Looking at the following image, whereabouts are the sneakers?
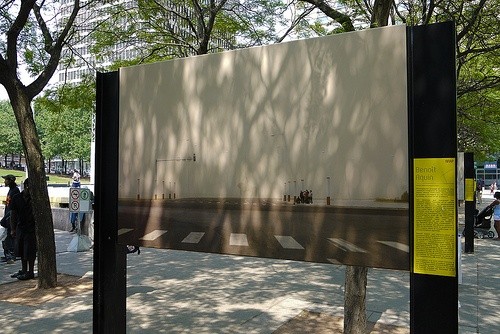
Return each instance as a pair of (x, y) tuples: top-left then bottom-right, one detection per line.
(10, 270), (34, 280)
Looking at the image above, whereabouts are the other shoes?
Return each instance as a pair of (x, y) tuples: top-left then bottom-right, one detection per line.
(7, 258), (15, 264)
(70, 227), (76, 232)
(1, 258), (8, 262)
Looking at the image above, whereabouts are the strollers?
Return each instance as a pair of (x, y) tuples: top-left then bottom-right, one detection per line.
(461, 203), (496, 239)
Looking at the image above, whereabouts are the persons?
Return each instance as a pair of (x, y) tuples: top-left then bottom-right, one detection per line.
(70, 192), (93, 233)
(476, 180), (485, 204)
(296, 189), (313, 204)
(491, 192), (500, 240)
(491, 182), (497, 193)
(0, 174), (37, 280)
(72, 169), (80, 188)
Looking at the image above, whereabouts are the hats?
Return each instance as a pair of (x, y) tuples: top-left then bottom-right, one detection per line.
(1, 174), (16, 181)
